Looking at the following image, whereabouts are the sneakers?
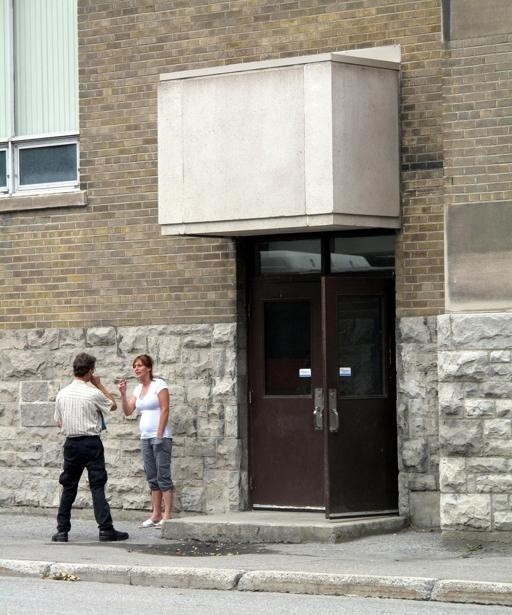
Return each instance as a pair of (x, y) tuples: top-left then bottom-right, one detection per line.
(138, 518), (166, 529)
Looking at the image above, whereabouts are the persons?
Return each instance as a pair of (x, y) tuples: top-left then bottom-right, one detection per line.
(51, 352), (130, 543)
(117, 354), (176, 530)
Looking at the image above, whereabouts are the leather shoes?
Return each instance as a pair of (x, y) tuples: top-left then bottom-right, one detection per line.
(98, 529), (131, 543)
(50, 530), (70, 542)
